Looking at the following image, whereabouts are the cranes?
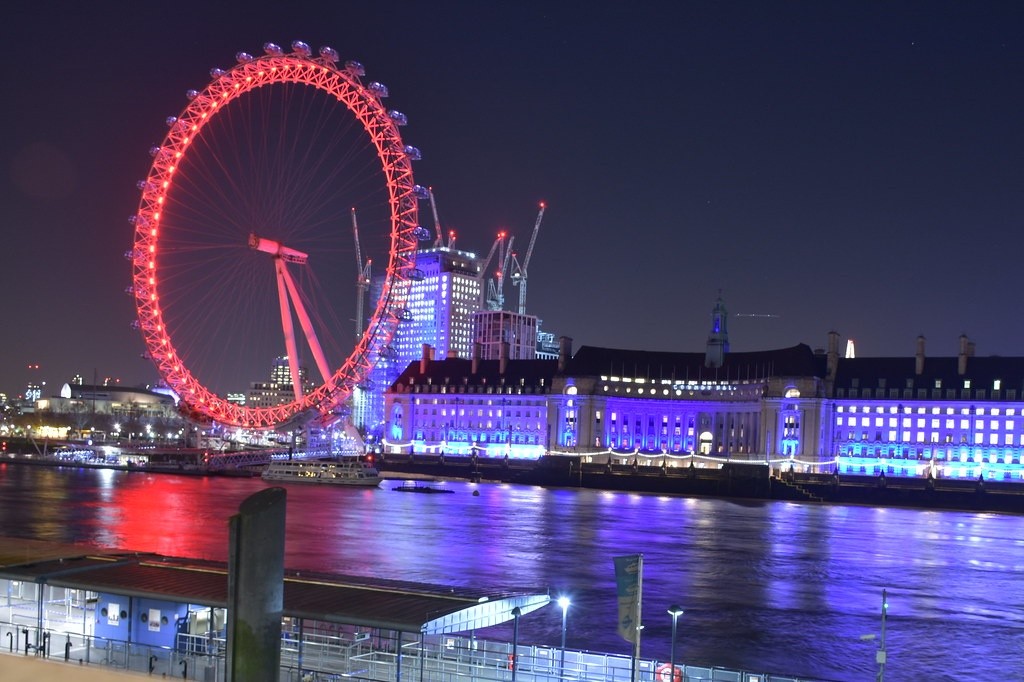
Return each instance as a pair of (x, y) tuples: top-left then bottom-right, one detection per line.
(350, 205), (372, 340)
(479, 201), (547, 314)
(429, 185), (457, 248)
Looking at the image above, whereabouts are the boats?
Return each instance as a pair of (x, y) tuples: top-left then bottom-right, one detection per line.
(260, 456), (384, 488)
(391, 480), (456, 495)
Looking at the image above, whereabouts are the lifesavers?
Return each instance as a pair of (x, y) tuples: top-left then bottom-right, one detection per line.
(656, 662), (681, 682)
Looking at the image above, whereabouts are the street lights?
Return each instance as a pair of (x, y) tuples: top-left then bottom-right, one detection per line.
(604, 445), (698, 476)
(557, 595), (573, 682)
(666, 605), (685, 682)
